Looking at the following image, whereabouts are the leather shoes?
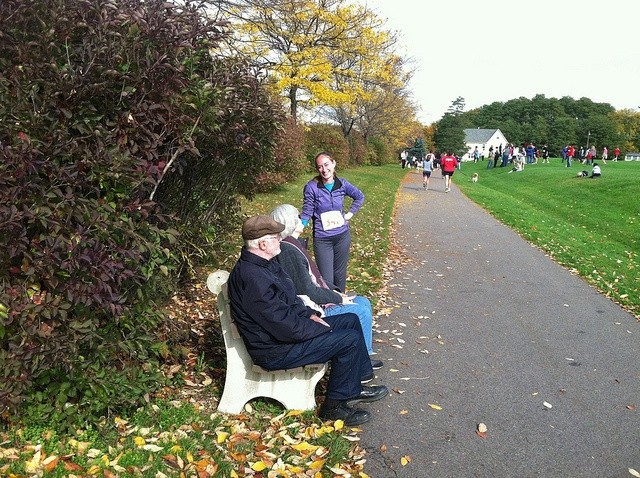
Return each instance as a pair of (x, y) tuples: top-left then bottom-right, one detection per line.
(345, 385), (388, 405)
(319, 403), (371, 426)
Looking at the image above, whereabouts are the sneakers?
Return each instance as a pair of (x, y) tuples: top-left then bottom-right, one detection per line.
(360, 373), (374, 384)
(370, 359), (383, 370)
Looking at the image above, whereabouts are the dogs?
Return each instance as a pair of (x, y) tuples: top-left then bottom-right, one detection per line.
(471, 172), (480, 183)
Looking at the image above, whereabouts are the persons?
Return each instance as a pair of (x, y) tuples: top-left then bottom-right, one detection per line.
(588, 162), (602, 178)
(487, 142), (550, 174)
(441, 151), (458, 193)
(615, 147), (620, 161)
(401, 150), (407, 169)
(297, 151), (365, 296)
(227, 216), (390, 427)
(562, 144), (596, 167)
(601, 146), (608, 164)
(270, 204), (384, 384)
(422, 154), (434, 190)
(425, 150), (436, 177)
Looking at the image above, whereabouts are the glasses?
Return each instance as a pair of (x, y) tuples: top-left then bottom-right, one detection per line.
(297, 214), (301, 218)
(272, 234), (282, 240)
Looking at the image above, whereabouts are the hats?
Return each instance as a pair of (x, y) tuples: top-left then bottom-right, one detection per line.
(242, 216), (285, 239)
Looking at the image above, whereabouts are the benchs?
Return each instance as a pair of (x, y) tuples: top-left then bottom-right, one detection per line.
(207, 268), (329, 417)
